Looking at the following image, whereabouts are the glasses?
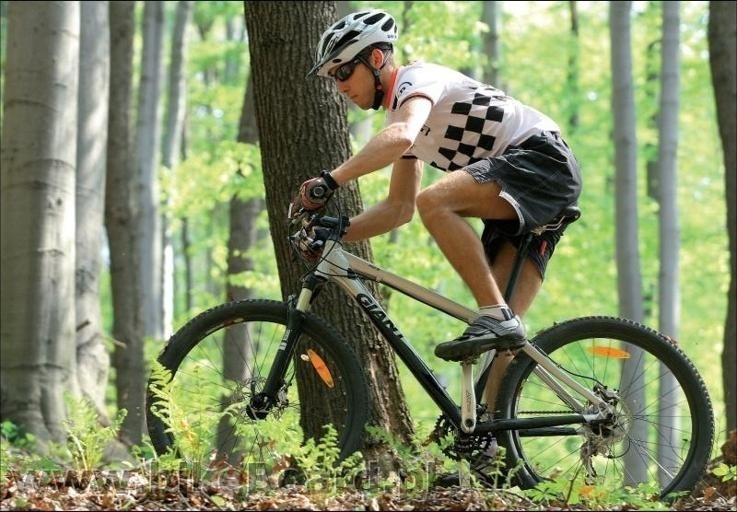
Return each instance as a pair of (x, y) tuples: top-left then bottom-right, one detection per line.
(335, 59), (360, 81)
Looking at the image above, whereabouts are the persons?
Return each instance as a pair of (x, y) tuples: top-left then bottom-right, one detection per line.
(297, 8), (585, 490)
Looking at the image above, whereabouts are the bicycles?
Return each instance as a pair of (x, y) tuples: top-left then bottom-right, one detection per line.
(145, 183), (716, 512)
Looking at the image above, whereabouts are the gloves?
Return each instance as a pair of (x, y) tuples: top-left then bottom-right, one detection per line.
(299, 239), (324, 264)
(298, 170), (340, 210)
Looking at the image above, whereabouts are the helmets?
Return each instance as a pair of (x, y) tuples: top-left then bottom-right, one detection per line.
(307, 9), (398, 77)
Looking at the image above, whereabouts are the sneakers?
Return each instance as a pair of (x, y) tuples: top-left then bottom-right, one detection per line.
(434, 314), (527, 362)
(441, 452), (517, 488)
